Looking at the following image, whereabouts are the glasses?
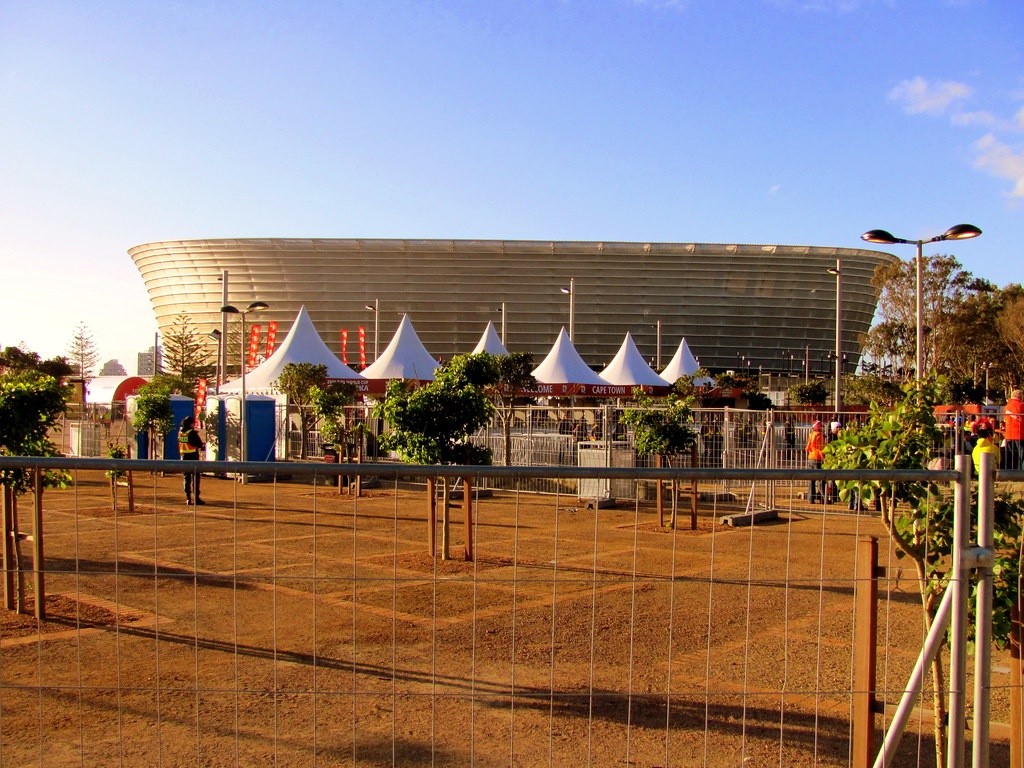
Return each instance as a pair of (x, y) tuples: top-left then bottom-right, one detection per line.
(966, 434), (971, 436)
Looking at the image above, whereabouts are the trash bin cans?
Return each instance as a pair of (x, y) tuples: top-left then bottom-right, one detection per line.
(69, 424), (101, 457)
(577, 440), (639, 502)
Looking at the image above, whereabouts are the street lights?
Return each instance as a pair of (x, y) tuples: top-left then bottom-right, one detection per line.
(208, 329), (222, 396)
(364, 304), (379, 363)
(219, 302), (269, 485)
(860, 223), (983, 408)
(560, 287), (575, 345)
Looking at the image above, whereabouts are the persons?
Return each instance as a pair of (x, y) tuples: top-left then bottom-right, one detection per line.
(176, 415), (206, 507)
(807, 389), (1024, 511)
(557, 411), (795, 465)
(101, 409), (113, 438)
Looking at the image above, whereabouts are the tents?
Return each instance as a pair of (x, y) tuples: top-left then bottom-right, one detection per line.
(598, 329), (667, 386)
(530, 325), (612, 385)
(218, 303), (368, 395)
(661, 337), (717, 388)
(471, 319), (509, 357)
(360, 312), (445, 380)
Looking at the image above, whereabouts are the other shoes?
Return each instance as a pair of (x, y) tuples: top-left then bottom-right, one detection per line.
(848, 502), (855, 510)
(820, 498), (824, 504)
(196, 500), (206, 505)
(809, 500), (816, 504)
(186, 500), (190, 505)
(856, 503), (868, 511)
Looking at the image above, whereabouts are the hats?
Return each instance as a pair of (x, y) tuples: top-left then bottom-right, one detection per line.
(831, 422), (841, 431)
(813, 420), (822, 429)
